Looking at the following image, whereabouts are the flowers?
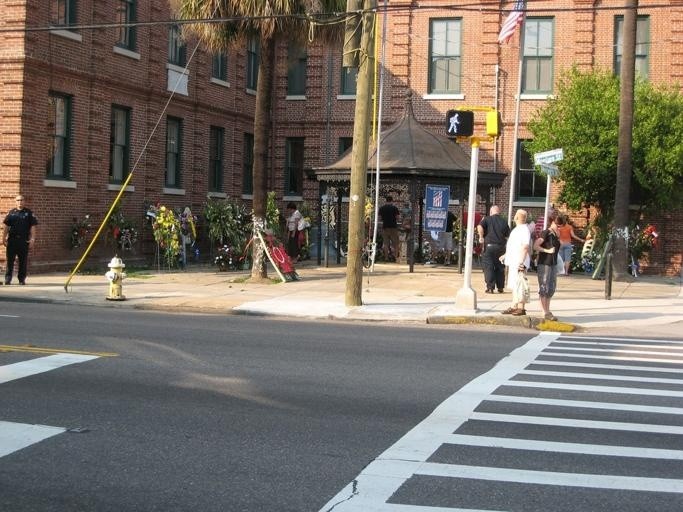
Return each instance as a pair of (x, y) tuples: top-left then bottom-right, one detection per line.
(143, 197), (201, 273)
(70, 213), (91, 249)
(201, 190), (320, 272)
(106, 203), (138, 252)
(570, 217), (661, 279)
(412, 237), (458, 266)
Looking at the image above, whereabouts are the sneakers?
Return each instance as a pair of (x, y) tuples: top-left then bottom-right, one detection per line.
(485, 288), (504, 293)
(19, 280), (25, 285)
(4, 280), (10, 285)
(545, 312), (558, 321)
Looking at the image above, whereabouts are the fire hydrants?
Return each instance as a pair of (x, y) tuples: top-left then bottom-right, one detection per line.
(105, 256), (126, 301)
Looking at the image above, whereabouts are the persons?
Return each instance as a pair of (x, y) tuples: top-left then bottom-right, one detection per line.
(476, 203), (585, 321)
(2, 195), (39, 285)
(285, 202), (305, 264)
(379, 196), (400, 262)
(425, 207), (457, 265)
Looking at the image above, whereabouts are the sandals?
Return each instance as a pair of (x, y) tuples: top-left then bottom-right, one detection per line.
(502, 307), (526, 315)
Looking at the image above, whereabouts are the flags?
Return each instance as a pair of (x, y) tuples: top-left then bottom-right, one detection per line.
(497, 0), (525, 44)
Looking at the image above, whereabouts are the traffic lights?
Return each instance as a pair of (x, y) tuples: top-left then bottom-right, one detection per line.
(445, 109), (473, 138)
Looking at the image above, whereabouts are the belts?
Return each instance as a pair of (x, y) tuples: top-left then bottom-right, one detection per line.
(487, 243), (502, 246)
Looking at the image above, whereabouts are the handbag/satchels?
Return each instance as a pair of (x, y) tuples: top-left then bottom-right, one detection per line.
(512, 271), (530, 304)
